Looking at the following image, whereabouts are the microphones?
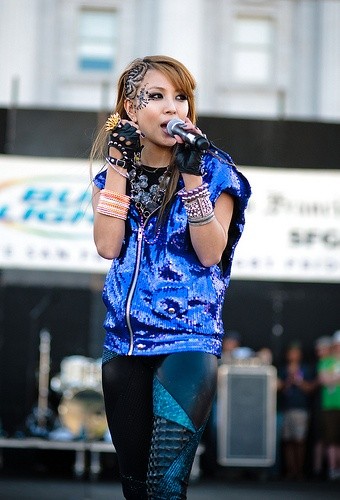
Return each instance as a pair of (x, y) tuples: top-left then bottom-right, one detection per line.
(166, 118), (209, 151)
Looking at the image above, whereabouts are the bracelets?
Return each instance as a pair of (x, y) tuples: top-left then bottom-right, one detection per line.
(176, 183), (215, 227)
(106, 158), (130, 179)
(96, 188), (130, 221)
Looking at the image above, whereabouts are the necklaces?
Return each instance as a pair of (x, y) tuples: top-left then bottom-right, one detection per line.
(130, 153), (172, 245)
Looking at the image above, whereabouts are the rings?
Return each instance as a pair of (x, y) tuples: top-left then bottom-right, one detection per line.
(105, 112), (122, 132)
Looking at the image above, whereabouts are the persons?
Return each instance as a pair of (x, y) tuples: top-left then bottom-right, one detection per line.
(45, 330), (340, 484)
(90, 56), (251, 500)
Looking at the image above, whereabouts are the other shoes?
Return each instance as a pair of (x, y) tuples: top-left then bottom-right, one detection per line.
(329, 470), (340, 480)
